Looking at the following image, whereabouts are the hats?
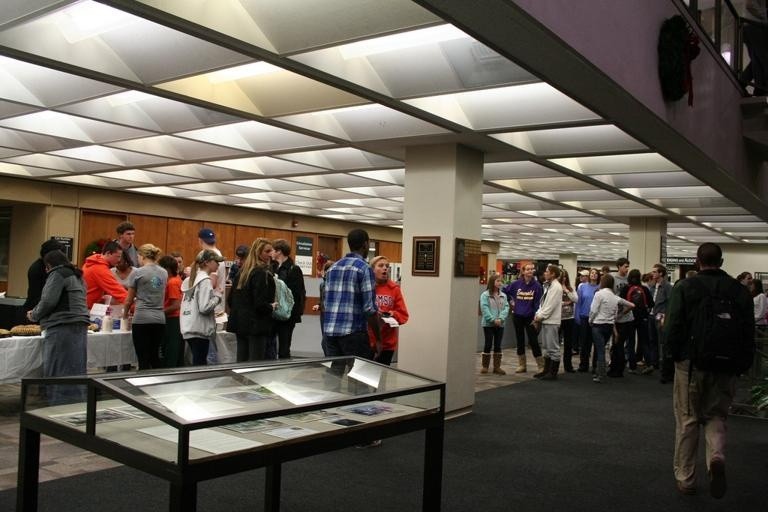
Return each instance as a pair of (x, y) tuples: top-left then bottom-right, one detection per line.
(196, 249), (226, 263)
(199, 229), (216, 243)
(579, 270), (590, 276)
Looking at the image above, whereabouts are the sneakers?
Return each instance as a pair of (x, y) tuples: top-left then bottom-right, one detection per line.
(579, 366), (588, 371)
(629, 369), (643, 375)
(565, 367), (574, 372)
(642, 366), (658, 374)
(356, 439), (380, 450)
(609, 371), (621, 378)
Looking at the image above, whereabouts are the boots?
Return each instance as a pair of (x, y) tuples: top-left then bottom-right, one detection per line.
(534, 356), (545, 373)
(540, 359), (560, 379)
(516, 354), (527, 372)
(533, 358), (553, 378)
(593, 363), (600, 379)
(593, 361), (608, 384)
(493, 354), (506, 374)
(480, 353), (490, 373)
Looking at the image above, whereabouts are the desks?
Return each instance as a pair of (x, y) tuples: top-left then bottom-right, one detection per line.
(1, 334), (236, 388)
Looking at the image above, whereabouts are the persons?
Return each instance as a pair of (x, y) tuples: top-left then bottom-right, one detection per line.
(80, 241), (136, 313)
(115, 222), (138, 270)
(481, 275), (510, 375)
(270, 240), (306, 358)
(576, 270), (590, 290)
(620, 270), (655, 374)
(642, 272), (656, 289)
(117, 243), (168, 371)
(601, 265), (610, 275)
(504, 263), (546, 373)
(159, 255), (183, 368)
(170, 252), (191, 280)
(558, 268), (578, 373)
(611, 258), (643, 375)
(575, 268), (601, 372)
(662, 243), (756, 498)
(589, 273), (635, 384)
(22, 241), (67, 309)
(184, 228), (227, 365)
(368, 256), (410, 365)
(737, 272), (768, 326)
(686, 271), (697, 279)
(535, 265), (563, 380)
(180, 249), (224, 364)
(226, 237), (279, 363)
(26, 249), (91, 404)
(109, 250), (137, 305)
(227, 244), (250, 283)
(320, 230), (382, 449)
(649, 264), (673, 330)
(311, 260), (335, 337)
(740, 0), (768, 96)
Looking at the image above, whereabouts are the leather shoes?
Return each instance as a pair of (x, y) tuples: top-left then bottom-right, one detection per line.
(677, 479), (699, 495)
(709, 458), (727, 499)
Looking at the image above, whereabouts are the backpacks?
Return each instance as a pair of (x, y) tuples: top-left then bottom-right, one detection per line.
(272, 272), (294, 321)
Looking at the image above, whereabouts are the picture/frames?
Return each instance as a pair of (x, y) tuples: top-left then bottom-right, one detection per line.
(412, 236), (441, 277)
